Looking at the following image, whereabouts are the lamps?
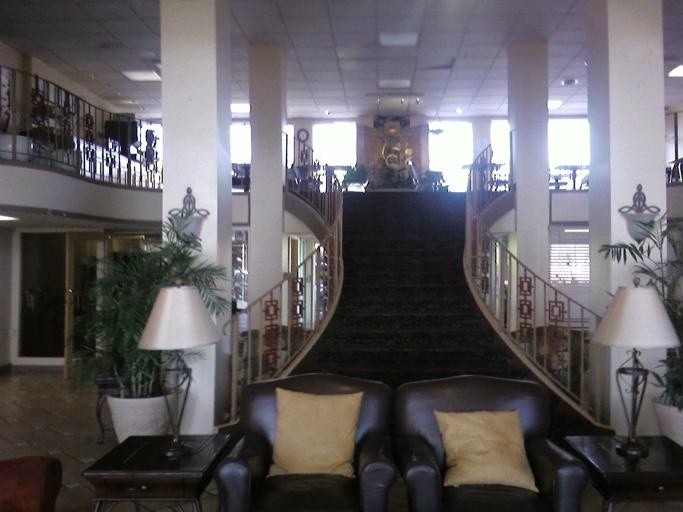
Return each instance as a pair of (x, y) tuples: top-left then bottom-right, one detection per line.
(592, 278), (680, 460)
(617, 181), (662, 246)
(137, 274), (221, 461)
(165, 184), (213, 247)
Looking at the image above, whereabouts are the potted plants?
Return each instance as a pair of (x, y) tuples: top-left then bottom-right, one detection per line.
(59, 216), (231, 447)
(598, 206), (682, 447)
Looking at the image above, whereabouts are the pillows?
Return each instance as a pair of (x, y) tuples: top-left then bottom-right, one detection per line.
(268, 388), (364, 480)
(434, 409), (540, 494)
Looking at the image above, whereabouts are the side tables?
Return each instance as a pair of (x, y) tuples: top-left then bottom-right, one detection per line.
(563, 430), (681, 512)
(81, 432), (235, 510)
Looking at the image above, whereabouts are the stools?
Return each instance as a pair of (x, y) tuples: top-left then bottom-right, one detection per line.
(0, 452), (64, 510)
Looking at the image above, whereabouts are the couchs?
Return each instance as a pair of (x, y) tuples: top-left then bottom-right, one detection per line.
(395, 375), (590, 511)
(216, 374), (394, 510)
